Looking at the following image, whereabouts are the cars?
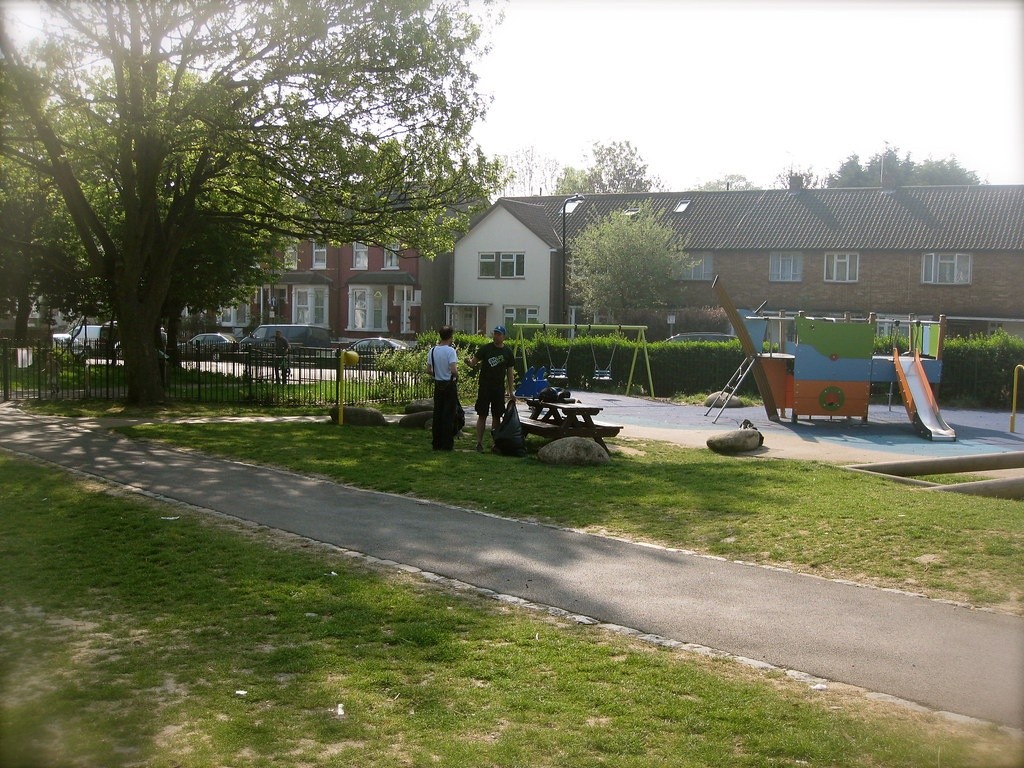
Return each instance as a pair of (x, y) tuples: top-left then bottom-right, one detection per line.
(113, 331), (167, 360)
(53, 333), (86, 360)
(176, 333), (238, 362)
(337, 337), (416, 372)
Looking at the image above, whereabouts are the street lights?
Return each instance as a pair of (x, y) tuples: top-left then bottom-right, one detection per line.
(563, 195), (586, 340)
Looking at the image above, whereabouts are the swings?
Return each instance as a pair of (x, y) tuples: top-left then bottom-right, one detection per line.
(590, 330), (620, 380)
(543, 331), (575, 380)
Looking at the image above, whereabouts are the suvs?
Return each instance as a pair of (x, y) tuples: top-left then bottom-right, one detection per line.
(660, 332), (738, 345)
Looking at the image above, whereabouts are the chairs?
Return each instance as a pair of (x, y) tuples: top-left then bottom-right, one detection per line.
(548, 368), (567, 378)
(593, 370), (613, 380)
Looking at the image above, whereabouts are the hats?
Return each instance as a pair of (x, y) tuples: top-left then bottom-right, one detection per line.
(493, 325), (506, 336)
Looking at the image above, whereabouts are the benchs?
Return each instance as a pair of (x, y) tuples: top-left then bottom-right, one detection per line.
(519, 417), (561, 441)
(562, 415), (624, 438)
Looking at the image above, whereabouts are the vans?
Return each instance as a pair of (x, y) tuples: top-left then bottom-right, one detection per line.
(238, 325), (338, 358)
(65, 324), (118, 360)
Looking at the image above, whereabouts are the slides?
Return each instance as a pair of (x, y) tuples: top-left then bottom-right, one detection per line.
(892, 348), (956, 441)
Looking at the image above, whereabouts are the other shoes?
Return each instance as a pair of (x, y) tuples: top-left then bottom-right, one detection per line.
(478, 444), (485, 454)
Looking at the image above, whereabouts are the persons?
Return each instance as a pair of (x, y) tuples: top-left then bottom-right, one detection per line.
(274, 330), (290, 386)
(161, 327), (167, 351)
(464, 326), (516, 454)
(425, 325), (460, 452)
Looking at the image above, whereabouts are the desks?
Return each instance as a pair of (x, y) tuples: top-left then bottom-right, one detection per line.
(526, 398), (610, 455)
